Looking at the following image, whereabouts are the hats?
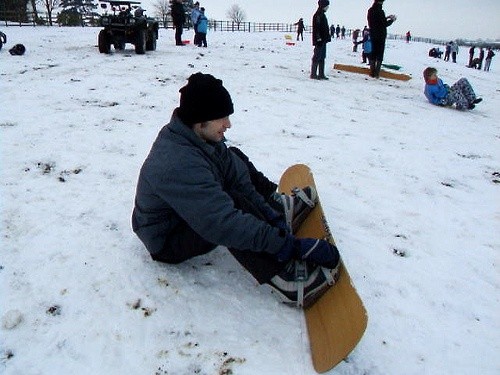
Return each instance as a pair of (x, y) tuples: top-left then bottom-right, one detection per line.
(179, 73), (234, 123)
(319, 0), (329, 8)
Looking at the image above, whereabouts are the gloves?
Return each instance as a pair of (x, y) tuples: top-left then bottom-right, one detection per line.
(265, 207), (290, 233)
(280, 235), (340, 268)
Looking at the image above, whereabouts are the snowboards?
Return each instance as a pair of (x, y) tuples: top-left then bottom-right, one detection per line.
(279, 163), (368, 372)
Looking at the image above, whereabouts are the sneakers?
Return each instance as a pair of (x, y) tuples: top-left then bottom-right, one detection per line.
(270, 185), (316, 234)
(264, 260), (340, 308)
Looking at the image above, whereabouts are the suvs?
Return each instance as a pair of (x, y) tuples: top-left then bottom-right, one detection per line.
(98, 0), (158, 55)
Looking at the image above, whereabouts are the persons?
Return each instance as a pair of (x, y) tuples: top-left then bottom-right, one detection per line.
(406, 30), (411, 43)
(353, 29), (373, 65)
(423, 67), (482, 110)
(477, 47), (484, 69)
(362, 26), (368, 49)
(190, 2), (200, 44)
(469, 46), (476, 66)
(429, 48), (437, 58)
(352, 29), (360, 52)
(132, 72), (341, 310)
(484, 46), (495, 71)
(341, 26), (345, 39)
(294, 18), (305, 41)
(194, 7), (208, 47)
(444, 42), (451, 62)
(434, 48), (441, 58)
(310, 0), (331, 80)
(449, 41), (459, 63)
(336, 24), (340, 37)
(468, 58), (480, 69)
(330, 25), (335, 38)
(171, 0), (186, 45)
